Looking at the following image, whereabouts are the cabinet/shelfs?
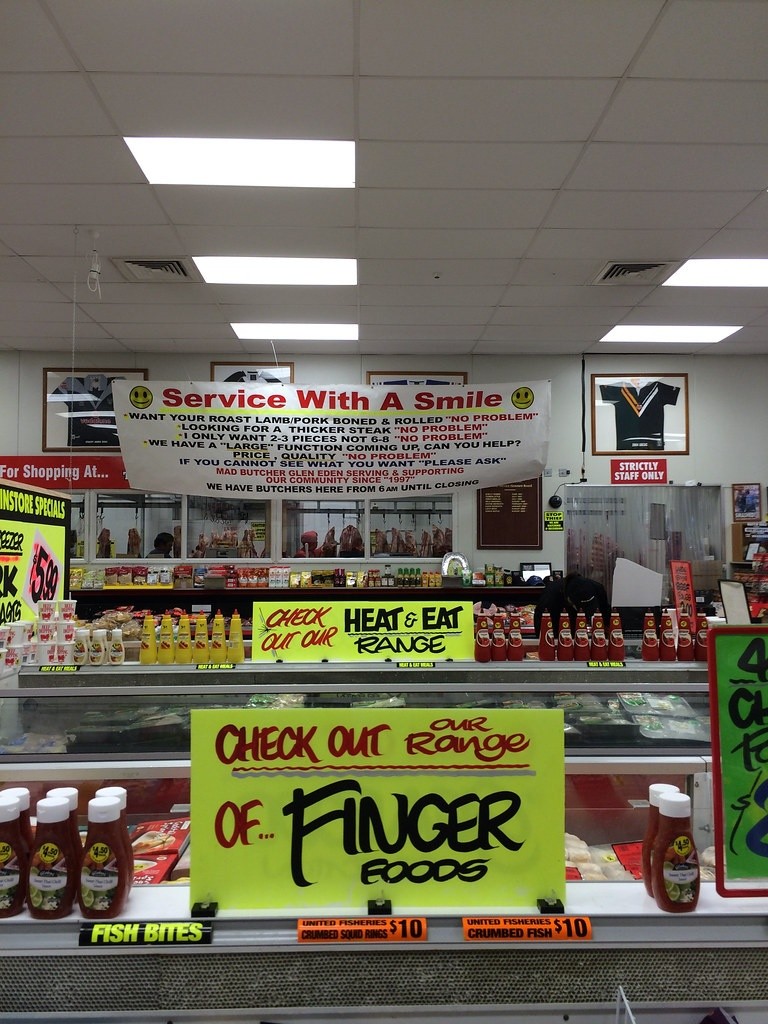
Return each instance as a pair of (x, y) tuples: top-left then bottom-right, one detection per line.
(731, 522), (768, 562)
(0, 662), (768, 1024)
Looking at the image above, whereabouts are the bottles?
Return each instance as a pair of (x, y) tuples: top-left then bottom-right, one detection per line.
(227, 609), (245, 663)
(268, 565), (290, 587)
(539, 607), (625, 662)
(138, 609), (157, 664)
(640, 607), (723, 662)
(76, 630), (125, 665)
(157, 609), (175, 664)
(641, 781), (700, 913)
(193, 610), (209, 664)
(210, 609), (226, 663)
(333, 564), (442, 587)
(175, 609), (192, 663)
(474, 608), (524, 663)
(0, 785), (135, 920)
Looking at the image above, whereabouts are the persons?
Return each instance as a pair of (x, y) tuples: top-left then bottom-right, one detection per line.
(295, 530), (323, 557)
(735, 487), (757, 513)
(145, 532), (174, 558)
(534, 575), (610, 638)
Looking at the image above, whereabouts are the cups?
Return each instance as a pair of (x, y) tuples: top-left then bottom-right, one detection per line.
(1, 600), (77, 674)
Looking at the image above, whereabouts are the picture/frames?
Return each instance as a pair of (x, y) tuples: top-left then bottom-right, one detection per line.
(732, 483), (761, 522)
(520, 562), (552, 583)
(717, 578), (752, 624)
(41, 368), (149, 451)
(590, 373), (690, 455)
(209, 361), (294, 383)
(366, 371), (468, 385)
(744, 541), (761, 562)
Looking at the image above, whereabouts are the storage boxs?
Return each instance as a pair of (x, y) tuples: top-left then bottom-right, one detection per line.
(512, 570), (523, 585)
(462, 692), (712, 749)
(203, 577), (226, 589)
(693, 571), (726, 591)
(553, 570), (563, 582)
(472, 580), (486, 587)
(651, 539), (687, 574)
(173, 577), (193, 588)
(692, 560), (722, 576)
(205, 546), (240, 558)
(65, 708), (190, 754)
(442, 576), (463, 587)
